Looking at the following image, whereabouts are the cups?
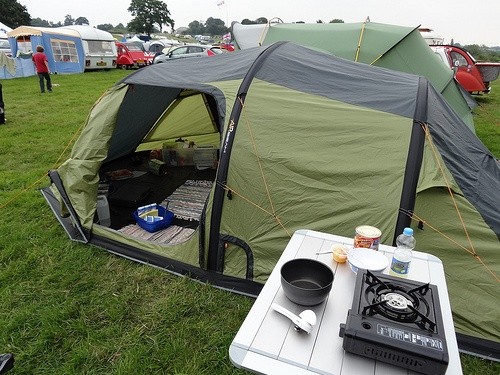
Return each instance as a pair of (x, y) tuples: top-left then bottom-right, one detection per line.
(333, 244), (348, 263)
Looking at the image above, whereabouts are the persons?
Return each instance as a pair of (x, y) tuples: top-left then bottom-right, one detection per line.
(32, 45), (53, 92)
(0, 84), (7, 124)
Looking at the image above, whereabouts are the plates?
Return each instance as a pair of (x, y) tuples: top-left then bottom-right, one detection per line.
(108, 175), (132, 180)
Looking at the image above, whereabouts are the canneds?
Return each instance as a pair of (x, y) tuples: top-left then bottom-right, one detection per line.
(352, 225), (382, 251)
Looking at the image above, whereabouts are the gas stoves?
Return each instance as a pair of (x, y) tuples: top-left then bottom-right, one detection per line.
(339, 269), (449, 375)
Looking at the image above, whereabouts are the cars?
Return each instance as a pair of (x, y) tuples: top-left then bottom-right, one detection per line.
(153, 44), (223, 64)
(0, 21), (211, 72)
(428, 44), (500, 96)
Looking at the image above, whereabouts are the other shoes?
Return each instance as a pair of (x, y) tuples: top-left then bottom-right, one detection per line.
(47, 88), (52, 91)
(0, 119), (7, 124)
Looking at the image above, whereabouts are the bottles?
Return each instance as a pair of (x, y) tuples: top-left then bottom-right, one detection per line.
(162, 142), (178, 167)
(147, 158), (168, 176)
(389, 227), (417, 279)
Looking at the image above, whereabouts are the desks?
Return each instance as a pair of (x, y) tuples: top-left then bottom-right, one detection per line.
(228, 228), (462, 375)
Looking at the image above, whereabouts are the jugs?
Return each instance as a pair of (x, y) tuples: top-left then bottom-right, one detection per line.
(96, 195), (111, 227)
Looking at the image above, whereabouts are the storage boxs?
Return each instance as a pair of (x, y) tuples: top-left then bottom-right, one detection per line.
(133, 204), (174, 232)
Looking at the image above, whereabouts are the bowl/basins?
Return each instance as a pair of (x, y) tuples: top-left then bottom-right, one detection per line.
(132, 205), (174, 233)
(280, 258), (335, 305)
(346, 248), (389, 274)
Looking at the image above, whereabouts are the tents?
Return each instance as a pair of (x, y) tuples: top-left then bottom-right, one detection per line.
(0, 22), (12, 56)
(40, 40), (500, 362)
(458, 83), (482, 107)
(126, 35), (145, 43)
(7, 26), (85, 75)
(231, 18), (478, 134)
